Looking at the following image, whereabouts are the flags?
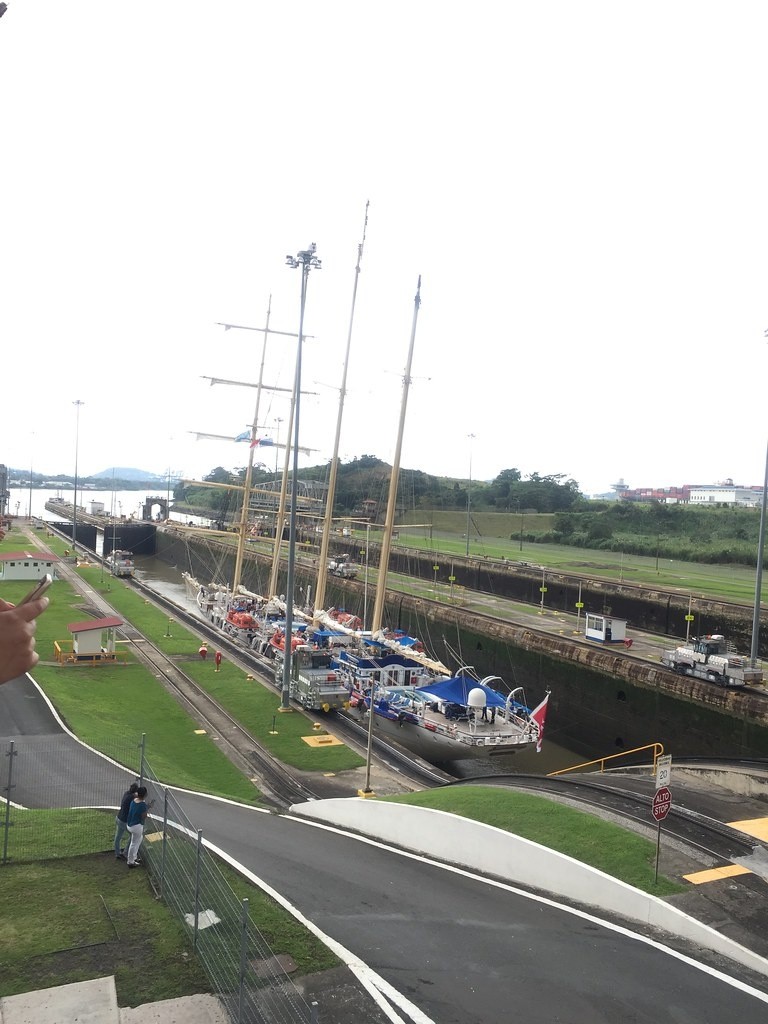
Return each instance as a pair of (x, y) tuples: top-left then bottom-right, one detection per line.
(232, 430), (274, 449)
(529, 694), (550, 753)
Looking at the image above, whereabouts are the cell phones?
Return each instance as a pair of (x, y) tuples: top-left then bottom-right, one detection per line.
(17, 574), (53, 607)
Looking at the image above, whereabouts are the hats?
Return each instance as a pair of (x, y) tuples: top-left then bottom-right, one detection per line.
(469, 707), (473, 710)
(138, 787), (148, 799)
(129, 783), (139, 793)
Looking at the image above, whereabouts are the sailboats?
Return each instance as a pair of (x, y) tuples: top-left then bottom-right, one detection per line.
(180, 196), (551, 761)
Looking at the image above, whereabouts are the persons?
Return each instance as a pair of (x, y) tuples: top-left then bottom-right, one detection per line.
(481, 705), (496, 725)
(115, 783), (154, 868)
(0, 597), (49, 685)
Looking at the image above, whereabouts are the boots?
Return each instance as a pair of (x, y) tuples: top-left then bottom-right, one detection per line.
(489, 719), (495, 724)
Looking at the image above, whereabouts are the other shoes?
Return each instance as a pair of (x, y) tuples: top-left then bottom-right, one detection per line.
(401, 725), (404, 728)
(116, 853), (128, 862)
(466, 718), (475, 723)
(481, 716), (489, 722)
(128, 859), (141, 867)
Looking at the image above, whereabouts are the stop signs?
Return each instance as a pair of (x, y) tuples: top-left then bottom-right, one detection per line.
(652, 789), (672, 820)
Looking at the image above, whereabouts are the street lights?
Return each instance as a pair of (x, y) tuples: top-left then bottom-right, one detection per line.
(465, 434), (478, 557)
(72, 399), (85, 549)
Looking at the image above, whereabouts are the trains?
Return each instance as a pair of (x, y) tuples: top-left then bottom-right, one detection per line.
(327, 553), (358, 579)
(661, 635), (764, 688)
(272, 644), (352, 713)
(110, 550), (136, 577)
